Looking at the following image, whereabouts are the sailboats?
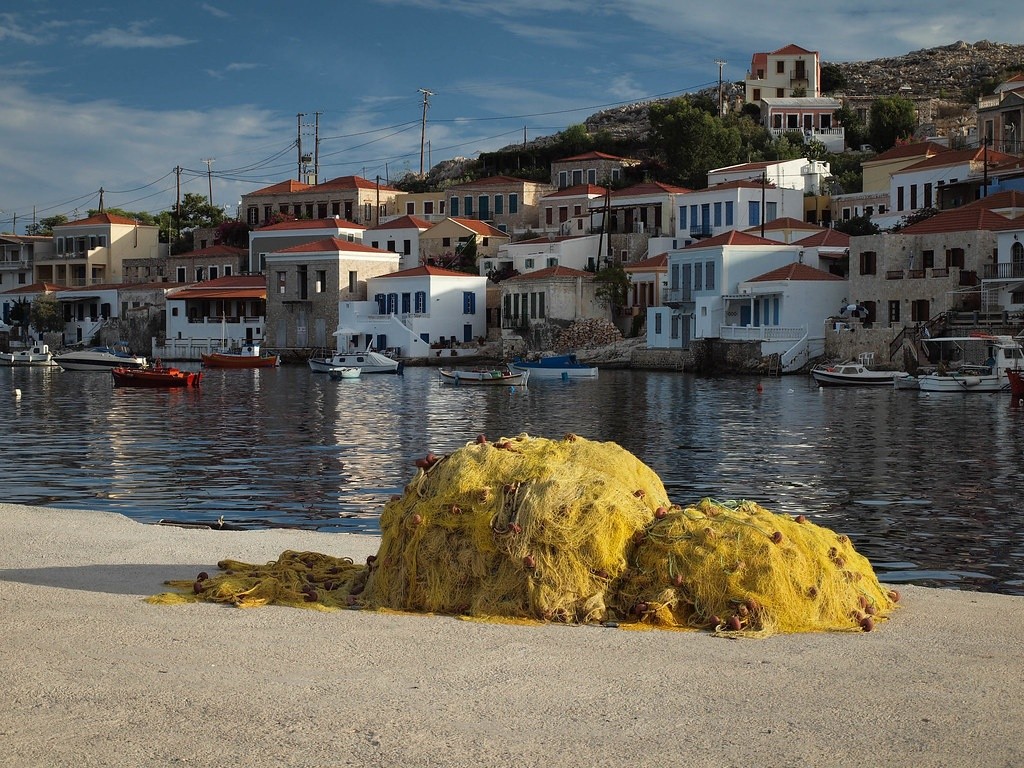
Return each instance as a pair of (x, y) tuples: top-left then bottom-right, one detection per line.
(201, 311), (277, 368)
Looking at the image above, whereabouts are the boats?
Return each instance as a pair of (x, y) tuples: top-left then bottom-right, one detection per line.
(439, 368), (526, 386)
(327, 367), (361, 378)
(111, 358), (203, 388)
(506, 354), (598, 380)
(917, 344), (1024, 392)
(1004, 368), (1024, 397)
(308, 351), (399, 373)
(810, 365), (909, 387)
(51, 347), (147, 371)
(0, 341), (58, 366)
